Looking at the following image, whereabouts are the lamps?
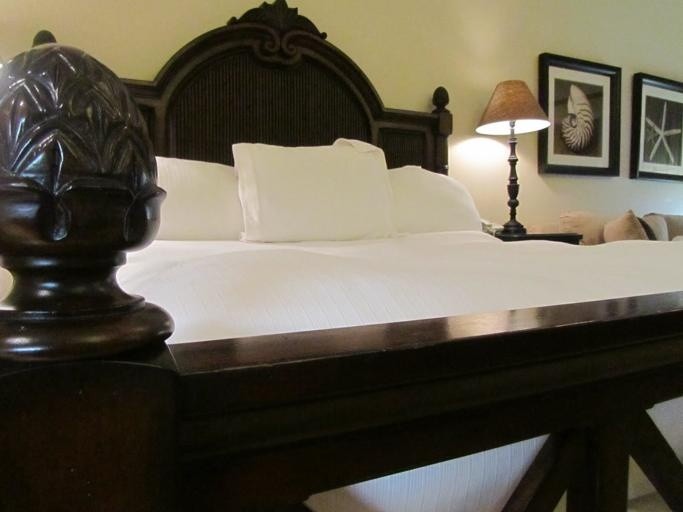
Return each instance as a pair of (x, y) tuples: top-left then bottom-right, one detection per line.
(475, 80), (551, 234)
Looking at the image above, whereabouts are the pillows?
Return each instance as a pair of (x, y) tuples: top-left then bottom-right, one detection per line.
(154, 156), (244, 241)
(231, 139), (410, 243)
(387, 165), (481, 235)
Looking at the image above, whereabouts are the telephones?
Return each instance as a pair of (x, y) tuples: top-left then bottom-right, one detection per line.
(481, 219), (504, 235)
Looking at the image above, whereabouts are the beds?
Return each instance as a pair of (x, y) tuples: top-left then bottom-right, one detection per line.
(0, 0), (683, 511)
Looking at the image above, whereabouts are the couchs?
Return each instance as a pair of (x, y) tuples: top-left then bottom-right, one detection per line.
(558, 211), (683, 246)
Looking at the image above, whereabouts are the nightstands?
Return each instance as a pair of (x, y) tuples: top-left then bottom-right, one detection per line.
(493, 232), (582, 246)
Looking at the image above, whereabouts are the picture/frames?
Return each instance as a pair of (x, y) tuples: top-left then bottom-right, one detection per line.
(538, 53), (683, 181)
